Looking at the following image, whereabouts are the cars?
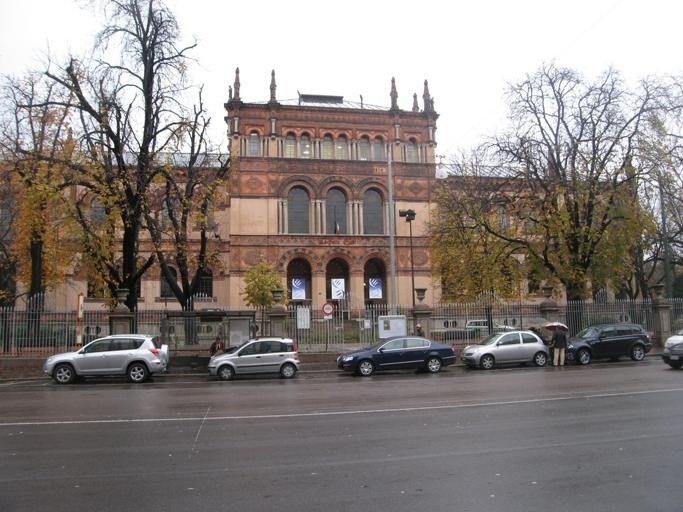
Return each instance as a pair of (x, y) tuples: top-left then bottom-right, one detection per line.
(465, 320), (514, 336)
(207, 337), (300, 380)
(336, 336), (457, 376)
(549, 323), (652, 365)
(460, 330), (550, 370)
(662, 329), (683, 368)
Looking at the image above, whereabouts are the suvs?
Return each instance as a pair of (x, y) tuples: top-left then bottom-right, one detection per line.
(42, 334), (168, 384)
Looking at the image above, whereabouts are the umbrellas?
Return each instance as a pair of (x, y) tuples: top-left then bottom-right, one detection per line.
(542, 319), (568, 331)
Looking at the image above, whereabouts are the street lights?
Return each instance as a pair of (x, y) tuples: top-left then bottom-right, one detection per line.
(399, 209), (416, 305)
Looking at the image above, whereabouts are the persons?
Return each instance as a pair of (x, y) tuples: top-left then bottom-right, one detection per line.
(413, 323), (425, 337)
(550, 325), (567, 367)
(209, 336), (224, 356)
